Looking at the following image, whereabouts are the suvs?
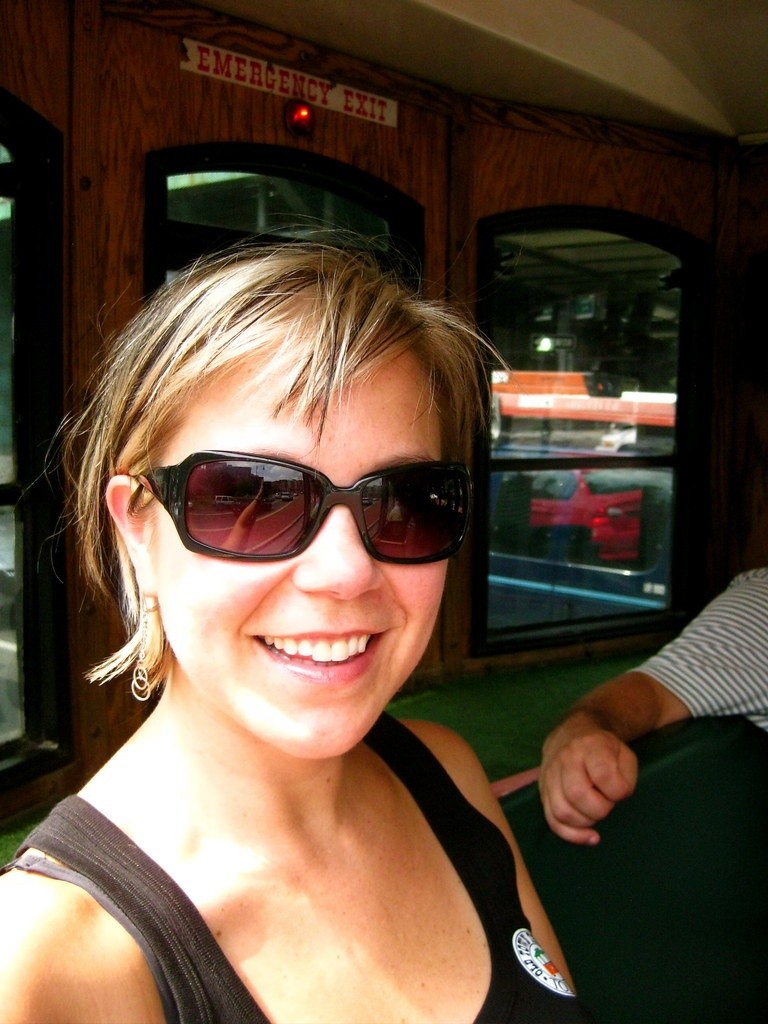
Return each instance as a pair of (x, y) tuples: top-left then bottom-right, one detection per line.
(214, 496), (242, 511)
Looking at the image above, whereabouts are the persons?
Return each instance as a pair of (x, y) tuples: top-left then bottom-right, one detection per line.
(0, 242), (588, 1024)
(222, 478), (266, 553)
(534, 568), (768, 845)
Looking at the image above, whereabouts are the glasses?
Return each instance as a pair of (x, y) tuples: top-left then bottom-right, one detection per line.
(129, 448), (476, 564)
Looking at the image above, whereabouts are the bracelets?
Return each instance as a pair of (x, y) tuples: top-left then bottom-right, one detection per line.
(254, 495), (262, 502)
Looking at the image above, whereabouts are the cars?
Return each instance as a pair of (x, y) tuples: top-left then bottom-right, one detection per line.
(492, 447), (645, 565)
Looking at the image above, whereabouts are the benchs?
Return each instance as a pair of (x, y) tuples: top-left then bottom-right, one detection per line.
(387, 644), (768, 1024)
